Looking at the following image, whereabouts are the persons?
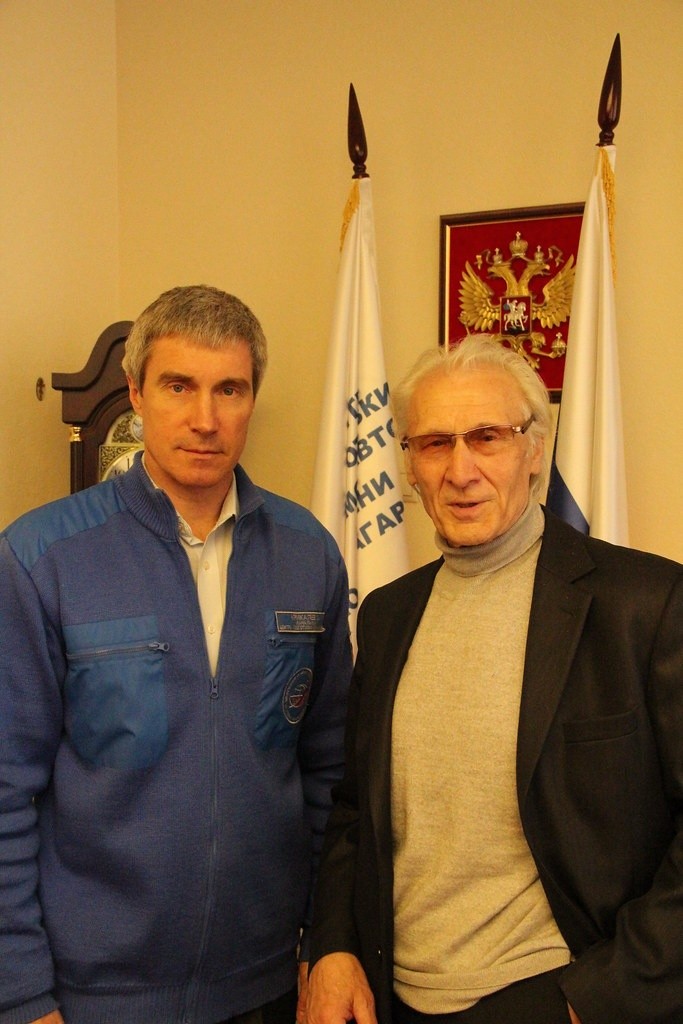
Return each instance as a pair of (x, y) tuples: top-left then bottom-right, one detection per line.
(0, 284), (358, 1024)
(301, 326), (682, 1024)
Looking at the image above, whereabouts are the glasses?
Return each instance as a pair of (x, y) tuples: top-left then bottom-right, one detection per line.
(401, 414), (536, 455)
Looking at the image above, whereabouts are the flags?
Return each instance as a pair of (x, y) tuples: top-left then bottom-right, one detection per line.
(541, 152), (643, 553)
(310, 176), (410, 670)
